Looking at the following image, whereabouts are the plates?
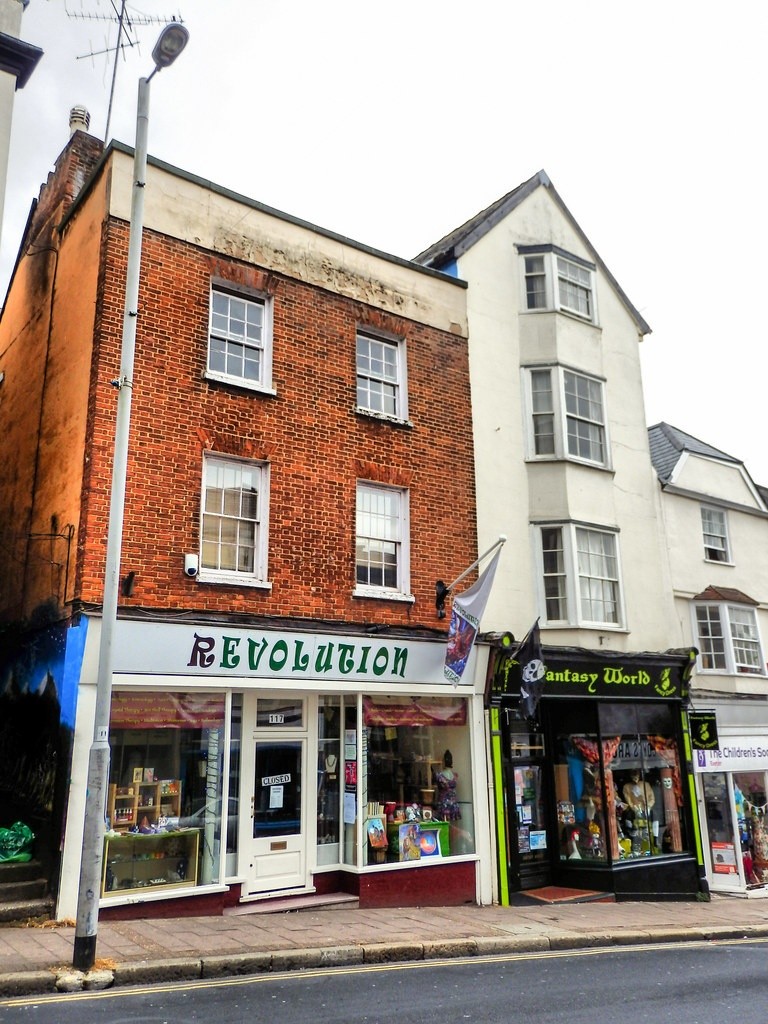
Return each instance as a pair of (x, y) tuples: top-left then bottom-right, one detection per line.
(149, 878), (166, 884)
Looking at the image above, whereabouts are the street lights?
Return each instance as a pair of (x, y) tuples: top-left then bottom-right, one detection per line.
(69, 21), (193, 971)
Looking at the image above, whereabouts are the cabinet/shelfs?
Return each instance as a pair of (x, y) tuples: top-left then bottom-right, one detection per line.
(368, 721), (444, 809)
(99, 825), (202, 899)
(106, 778), (184, 835)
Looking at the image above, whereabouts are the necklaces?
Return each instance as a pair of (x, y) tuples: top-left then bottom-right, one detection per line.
(326, 758), (335, 767)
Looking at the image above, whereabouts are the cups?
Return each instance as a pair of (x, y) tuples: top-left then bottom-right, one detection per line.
(138, 795), (144, 806)
(147, 798), (153, 806)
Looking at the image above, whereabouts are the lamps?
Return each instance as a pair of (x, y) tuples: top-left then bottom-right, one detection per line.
(476, 630), (516, 649)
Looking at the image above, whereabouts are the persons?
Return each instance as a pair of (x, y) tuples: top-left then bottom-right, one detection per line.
(623, 770), (655, 839)
(436, 766), (462, 822)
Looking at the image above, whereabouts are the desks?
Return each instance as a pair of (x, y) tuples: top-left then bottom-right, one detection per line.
(384, 820), (450, 862)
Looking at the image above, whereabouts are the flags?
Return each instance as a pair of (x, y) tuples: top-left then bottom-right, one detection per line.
(444, 544), (503, 688)
(511, 621), (548, 721)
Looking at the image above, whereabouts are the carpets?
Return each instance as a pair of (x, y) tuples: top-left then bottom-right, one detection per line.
(518, 885), (606, 904)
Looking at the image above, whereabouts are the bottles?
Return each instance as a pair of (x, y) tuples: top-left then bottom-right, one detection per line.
(115, 808), (133, 821)
